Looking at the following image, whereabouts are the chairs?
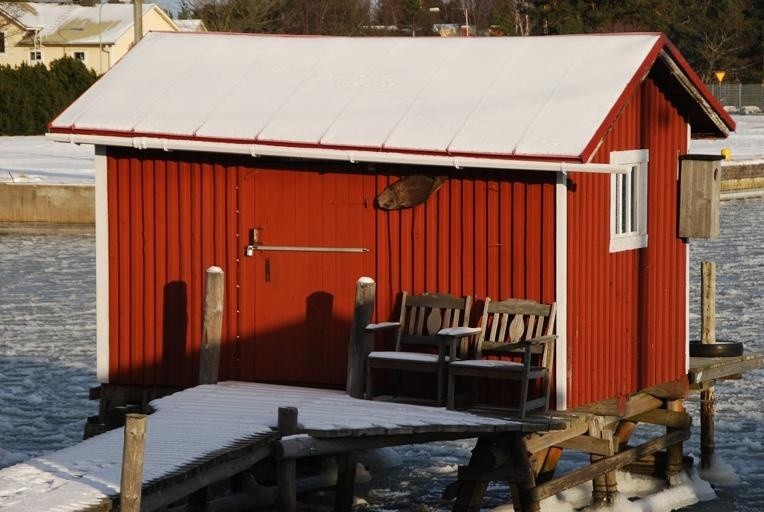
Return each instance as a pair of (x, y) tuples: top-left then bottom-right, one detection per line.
(364, 289), (557, 418)
